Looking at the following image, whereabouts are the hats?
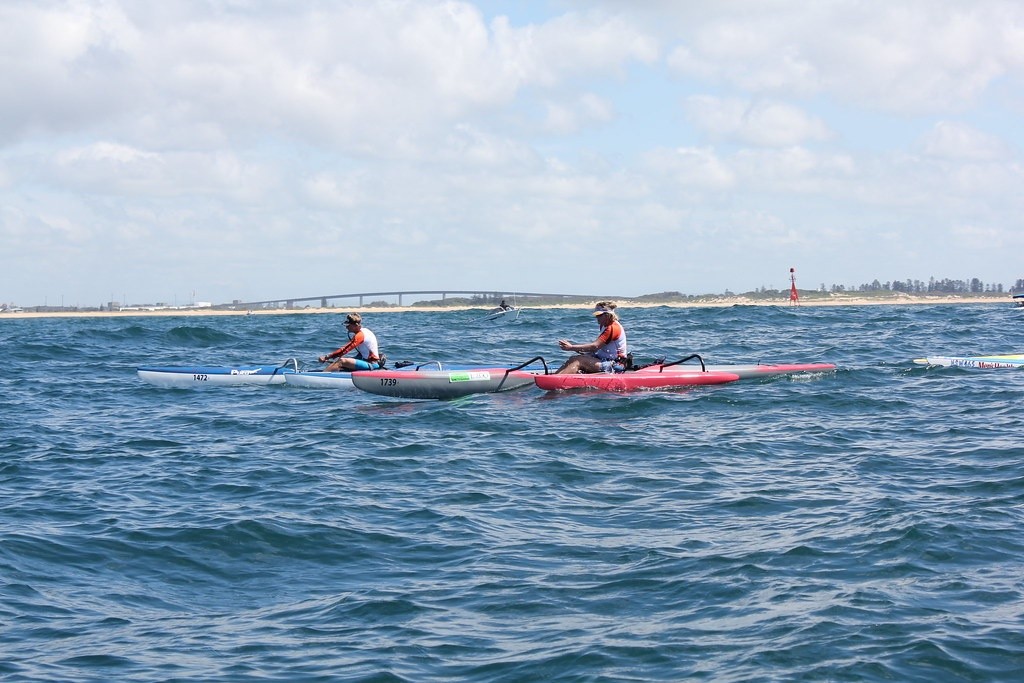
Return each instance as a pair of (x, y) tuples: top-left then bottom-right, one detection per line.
(592, 304), (614, 316)
(343, 318), (360, 324)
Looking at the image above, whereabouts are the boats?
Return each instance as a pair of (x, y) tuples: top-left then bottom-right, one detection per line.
(351, 360), (539, 398)
(535, 354), (739, 391)
(135, 357), (301, 386)
(914, 353), (1024, 369)
(1008, 294), (1024, 312)
(283, 371), (357, 390)
(471, 305), (523, 323)
(641, 361), (836, 380)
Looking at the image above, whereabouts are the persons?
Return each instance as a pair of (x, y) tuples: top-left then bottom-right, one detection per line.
(500, 300), (511, 311)
(319, 313), (380, 372)
(553, 302), (628, 374)
